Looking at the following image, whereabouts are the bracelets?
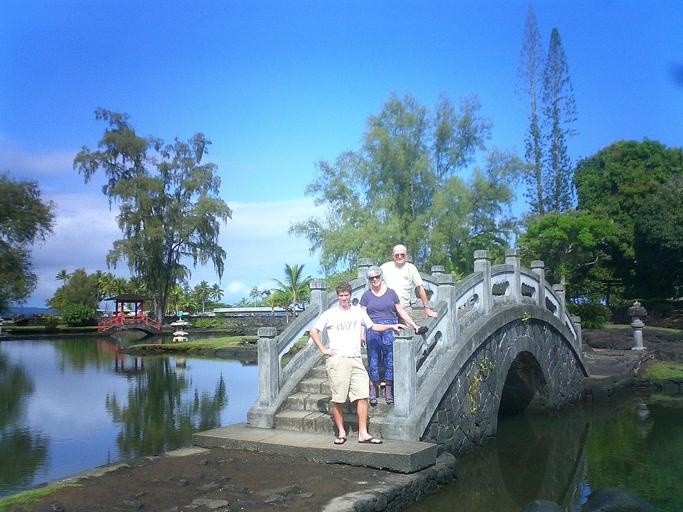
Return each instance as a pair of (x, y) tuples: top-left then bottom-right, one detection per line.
(424, 305), (430, 308)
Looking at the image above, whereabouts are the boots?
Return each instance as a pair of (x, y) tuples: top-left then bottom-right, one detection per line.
(368, 381), (379, 406)
(385, 380), (394, 403)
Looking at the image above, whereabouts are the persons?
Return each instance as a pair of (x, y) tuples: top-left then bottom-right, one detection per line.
(357, 265), (424, 406)
(307, 280), (408, 445)
(377, 244), (439, 329)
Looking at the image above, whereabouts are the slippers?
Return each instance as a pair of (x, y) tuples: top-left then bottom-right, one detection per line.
(333, 436), (347, 444)
(357, 437), (382, 445)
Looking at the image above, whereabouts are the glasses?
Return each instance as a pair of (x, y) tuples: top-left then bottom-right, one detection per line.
(394, 253), (406, 259)
(368, 274), (379, 281)
